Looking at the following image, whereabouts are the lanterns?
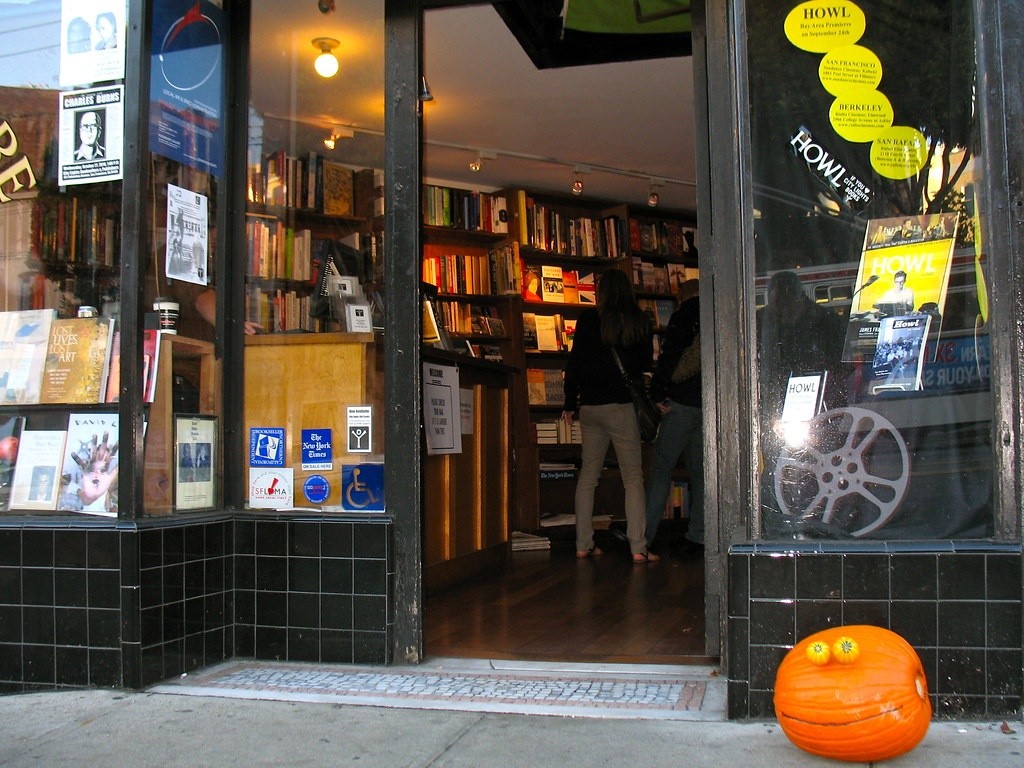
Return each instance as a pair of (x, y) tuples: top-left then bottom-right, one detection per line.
(773, 623), (932, 762)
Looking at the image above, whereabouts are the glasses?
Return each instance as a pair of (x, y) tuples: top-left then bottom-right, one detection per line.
(79, 123), (101, 130)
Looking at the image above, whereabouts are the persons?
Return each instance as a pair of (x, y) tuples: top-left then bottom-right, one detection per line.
(758, 271), (847, 526)
(180, 443), (210, 482)
(864, 211), (951, 379)
(29, 465), (54, 500)
(560, 268), (663, 563)
(609, 279), (705, 546)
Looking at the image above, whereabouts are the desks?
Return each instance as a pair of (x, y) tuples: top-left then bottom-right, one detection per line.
(838, 393), (991, 527)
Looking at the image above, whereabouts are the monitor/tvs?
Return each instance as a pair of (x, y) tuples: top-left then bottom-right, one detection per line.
(308, 239), (372, 319)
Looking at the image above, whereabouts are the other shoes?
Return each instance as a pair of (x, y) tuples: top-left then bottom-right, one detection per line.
(607, 523), (630, 542)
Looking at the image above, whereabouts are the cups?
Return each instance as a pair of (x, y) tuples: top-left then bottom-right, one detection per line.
(77, 306), (99, 319)
(151, 294), (180, 336)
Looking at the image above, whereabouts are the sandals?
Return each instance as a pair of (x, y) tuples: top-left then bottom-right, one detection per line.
(633, 550), (662, 562)
(577, 544), (606, 556)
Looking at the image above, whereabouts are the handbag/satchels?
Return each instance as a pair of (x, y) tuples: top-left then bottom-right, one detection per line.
(670, 334), (700, 383)
(627, 376), (663, 444)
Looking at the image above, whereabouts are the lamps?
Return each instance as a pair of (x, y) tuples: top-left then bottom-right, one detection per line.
(310, 38), (340, 78)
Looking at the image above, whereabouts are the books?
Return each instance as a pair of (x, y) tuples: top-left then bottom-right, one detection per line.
(781, 370), (828, 436)
(8, 89), (777, 459)
(512, 464), (692, 552)
(868, 314), (933, 395)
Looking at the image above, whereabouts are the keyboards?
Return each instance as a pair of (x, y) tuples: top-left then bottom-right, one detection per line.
(283, 329), (317, 333)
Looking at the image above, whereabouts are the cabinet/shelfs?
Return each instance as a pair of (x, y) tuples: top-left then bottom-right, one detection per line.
(238, 335), (522, 592)
(0, 113), (701, 516)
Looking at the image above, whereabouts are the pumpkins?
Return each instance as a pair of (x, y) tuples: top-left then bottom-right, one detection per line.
(774, 625), (931, 761)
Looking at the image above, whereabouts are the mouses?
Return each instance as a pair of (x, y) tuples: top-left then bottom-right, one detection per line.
(255, 328), (268, 334)
